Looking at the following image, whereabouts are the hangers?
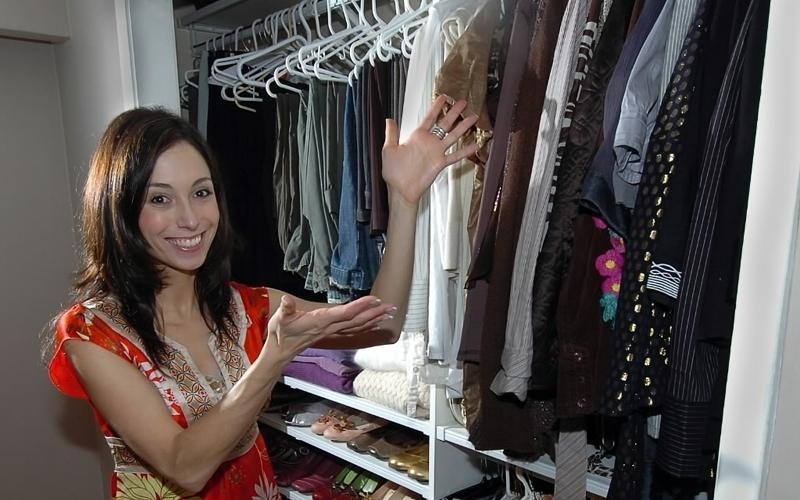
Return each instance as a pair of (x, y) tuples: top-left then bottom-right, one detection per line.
(181, 0), (465, 114)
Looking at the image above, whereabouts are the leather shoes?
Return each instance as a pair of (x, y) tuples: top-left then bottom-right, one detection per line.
(283, 400), (337, 427)
(387, 441), (430, 473)
(310, 408), (347, 437)
(274, 450), (323, 488)
(366, 428), (425, 464)
(346, 424), (404, 452)
(322, 417), (387, 443)
(408, 460), (429, 483)
(282, 395), (320, 420)
(290, 461), (340, 493)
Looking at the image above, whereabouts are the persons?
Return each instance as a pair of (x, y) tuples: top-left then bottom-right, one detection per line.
(47, 93), (481, 499)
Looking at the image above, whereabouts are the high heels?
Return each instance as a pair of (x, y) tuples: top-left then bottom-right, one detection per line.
(388, 487), (415, 500)
(368, 479), (395, 500)
(332, 471), (385, 499)
(312, 463), (361, 500)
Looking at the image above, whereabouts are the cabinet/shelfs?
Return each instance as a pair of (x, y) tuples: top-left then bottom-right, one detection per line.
(171, 0), (799, 499)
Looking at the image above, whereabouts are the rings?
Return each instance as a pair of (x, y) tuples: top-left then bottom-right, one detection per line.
(432, 127), (446, 140)
